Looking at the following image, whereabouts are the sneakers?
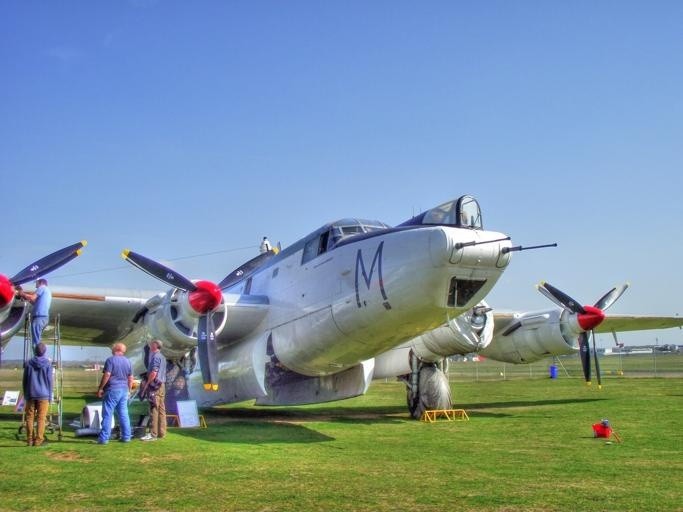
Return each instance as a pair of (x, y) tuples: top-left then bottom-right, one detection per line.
(140, 434), (157, 442)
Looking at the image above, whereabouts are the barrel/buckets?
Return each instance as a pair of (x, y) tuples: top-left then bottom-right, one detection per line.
(593, 423), (613, 437)
(551, 366), (558, 378)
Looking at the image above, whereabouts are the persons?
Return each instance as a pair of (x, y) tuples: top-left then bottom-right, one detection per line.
(138, 339), (167, 441)
(18, 278), (51, 355)
(95, 343), (133, 445)
(259, 236), (271, 253)
(167, 375), (188, 414)
(21, 343), (53, 446)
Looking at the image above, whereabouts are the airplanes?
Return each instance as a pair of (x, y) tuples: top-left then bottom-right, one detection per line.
(0, 196), (681, 419)
